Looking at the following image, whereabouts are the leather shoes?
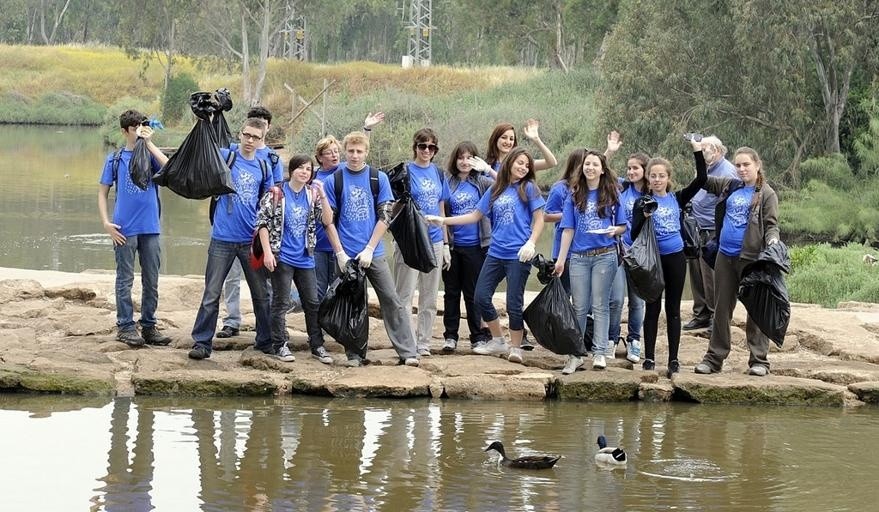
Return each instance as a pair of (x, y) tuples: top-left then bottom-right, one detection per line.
(683, 317), (711, 331)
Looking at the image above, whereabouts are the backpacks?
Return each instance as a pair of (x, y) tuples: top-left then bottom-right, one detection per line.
(209, 149), (267, 226)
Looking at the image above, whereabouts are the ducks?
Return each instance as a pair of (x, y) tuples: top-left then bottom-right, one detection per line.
(484, 441), (561, 469)
(594, 436), (627, 465)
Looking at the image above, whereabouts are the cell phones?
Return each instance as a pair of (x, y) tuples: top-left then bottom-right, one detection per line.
(685, 133), (703, 142)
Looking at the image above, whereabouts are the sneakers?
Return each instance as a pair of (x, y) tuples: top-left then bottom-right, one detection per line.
(404, 345), (431, 367)
(442, 333), (535, 365)
(560, 350), (608, 374)
(188, 343), (212, 359)
(310, 346), (334, 365)
(626, 339), (658, 371)
(694, 362), (712, 375)
(748, 363), (768, 376)
(667, 360), (679, 379)
(257, 344), (296, 362)
(216, 325), (240, 338)
(142, 326), (173, 346)
(115, 326), (145, 346)
(346, 357), (363, 367)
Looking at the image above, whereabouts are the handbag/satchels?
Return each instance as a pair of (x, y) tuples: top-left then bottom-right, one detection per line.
(249, 185), (282, 271)
(677, 191), (702, 259)
(701, 178), (742, 270)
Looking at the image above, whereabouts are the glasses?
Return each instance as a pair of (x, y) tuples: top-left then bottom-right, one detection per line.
(242, 131), (262, 142)
(416, 142), (438, 153)
